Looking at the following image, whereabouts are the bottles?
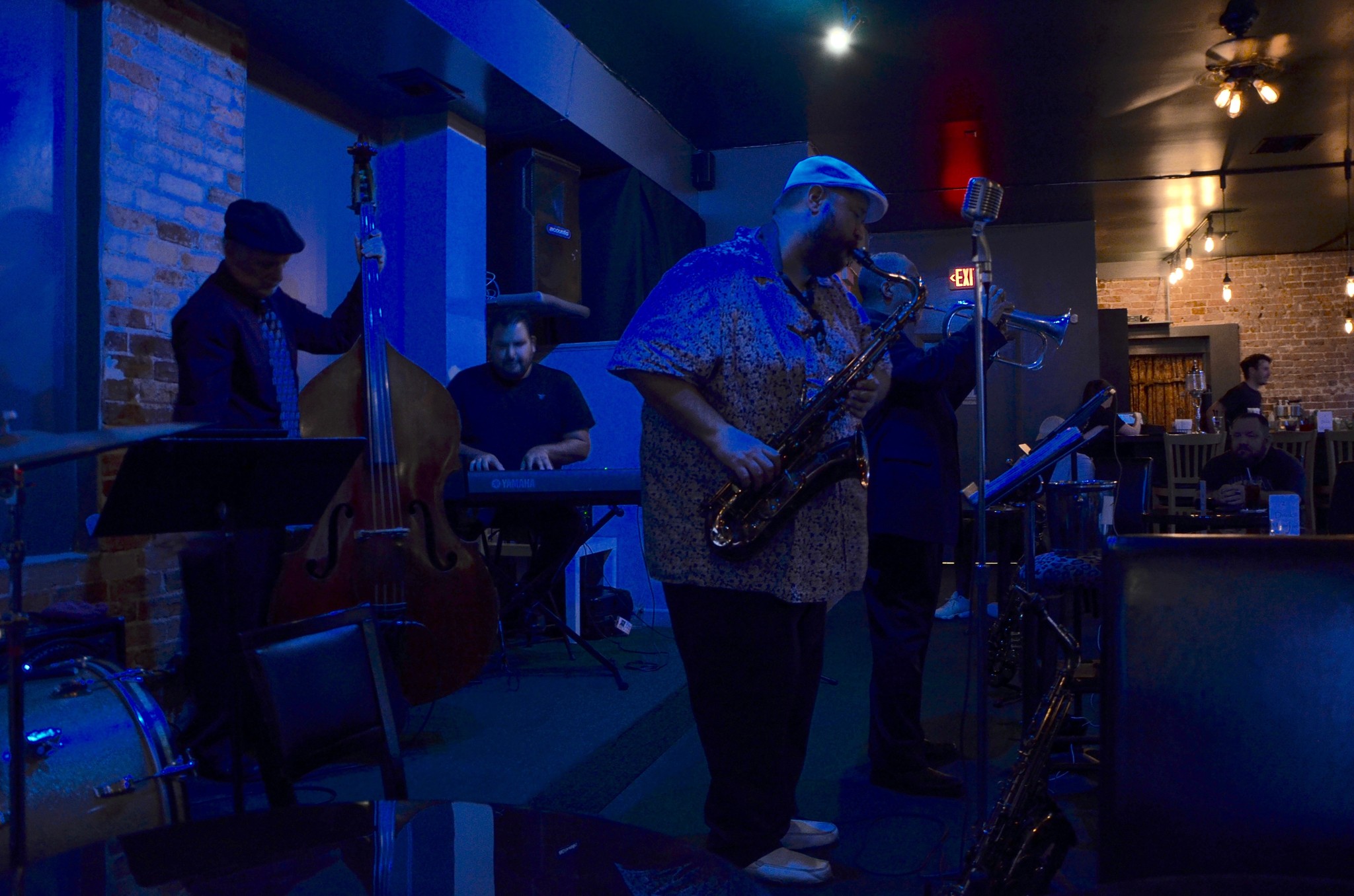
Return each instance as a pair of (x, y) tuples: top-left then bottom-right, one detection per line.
(2, 409), (19, 433)
(1276, 399), (1301, 417)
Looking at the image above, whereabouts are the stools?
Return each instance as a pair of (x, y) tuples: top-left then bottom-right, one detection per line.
(488, 533), (620, 645)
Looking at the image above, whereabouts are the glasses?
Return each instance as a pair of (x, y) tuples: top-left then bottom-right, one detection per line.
(887, 275), (924, 291)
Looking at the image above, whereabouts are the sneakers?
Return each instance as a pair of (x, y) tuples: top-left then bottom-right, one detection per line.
(933, 591), (970, 621)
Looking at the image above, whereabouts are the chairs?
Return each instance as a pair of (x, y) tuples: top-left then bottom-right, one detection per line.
(231, 602), (410, 822)
(1012, 474), (1119, 657)
(1270, 430), (1319, 533)
(1153, 430), (1228, 532)
(1094, 456), (1153, 535)
(1314, 428), (1354, 509)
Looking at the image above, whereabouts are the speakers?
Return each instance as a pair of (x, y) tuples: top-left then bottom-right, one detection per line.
(691, 150), (716, 191)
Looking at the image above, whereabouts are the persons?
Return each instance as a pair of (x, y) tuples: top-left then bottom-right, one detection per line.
(1206, 353), (1273, 450)
(171, 199), (387, 783)
(932, 416), (1066, 620)
(444, 310), (595, 637)
(606, 148), (890, 881)
(859, 250), (1017, 800)
(1076, 378), (1142, 458)
(1191, 413), (1306, 512)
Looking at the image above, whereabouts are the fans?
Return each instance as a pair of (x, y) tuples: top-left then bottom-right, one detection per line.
(1109, 0), (1354, 123)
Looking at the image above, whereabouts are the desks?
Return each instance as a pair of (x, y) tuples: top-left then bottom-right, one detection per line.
(0, 798), (776, 896)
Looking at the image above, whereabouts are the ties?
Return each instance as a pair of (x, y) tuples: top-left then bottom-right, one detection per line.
(257, 299), (302, 439)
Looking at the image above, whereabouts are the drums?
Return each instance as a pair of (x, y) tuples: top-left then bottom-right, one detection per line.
(0, 651), (191, 866)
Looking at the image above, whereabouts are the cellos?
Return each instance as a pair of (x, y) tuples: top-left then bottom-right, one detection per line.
(294, 135), (505, 753)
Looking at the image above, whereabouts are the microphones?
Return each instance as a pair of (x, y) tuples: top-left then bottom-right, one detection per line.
(961, 178), (1004, 293)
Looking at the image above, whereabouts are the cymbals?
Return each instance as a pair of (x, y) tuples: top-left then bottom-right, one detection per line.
(0, 416), (201, 474)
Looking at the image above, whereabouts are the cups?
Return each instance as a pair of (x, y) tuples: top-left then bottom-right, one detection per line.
(1244, 481), (1262, 510)
(1212, 417), (1223, 433)
(1283, 421), (1297, 431)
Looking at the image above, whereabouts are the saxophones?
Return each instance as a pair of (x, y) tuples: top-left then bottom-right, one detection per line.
(954, 583), (1088, 896)
(701, 245), (928, 562)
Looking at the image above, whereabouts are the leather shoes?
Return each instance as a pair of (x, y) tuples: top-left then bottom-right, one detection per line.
(741, 848), (834, 889)
(916, 738), (959, 765)
(869, 763), (965, 799)
(776, 819), (839, 853)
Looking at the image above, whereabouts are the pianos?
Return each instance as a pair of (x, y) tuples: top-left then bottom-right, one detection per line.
(447, 467), (642, 689)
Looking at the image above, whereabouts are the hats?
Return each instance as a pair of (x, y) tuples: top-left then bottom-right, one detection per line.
(784, 154), (889, 226)
(223, 198), (306, 256)
(1035, 414), (1068, 441)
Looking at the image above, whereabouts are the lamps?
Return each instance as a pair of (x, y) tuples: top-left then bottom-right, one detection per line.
(1185, 358), (1211, 434)
(1213, 62), (1282, 119)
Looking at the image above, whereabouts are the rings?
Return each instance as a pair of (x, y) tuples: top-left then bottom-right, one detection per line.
(1235, 490), (1241, 494)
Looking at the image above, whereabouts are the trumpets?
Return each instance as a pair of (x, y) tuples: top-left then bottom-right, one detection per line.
(918, 294), (1075, 376)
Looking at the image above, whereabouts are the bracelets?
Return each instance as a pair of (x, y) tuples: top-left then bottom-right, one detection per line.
(1244, 483), (1259, 507)
